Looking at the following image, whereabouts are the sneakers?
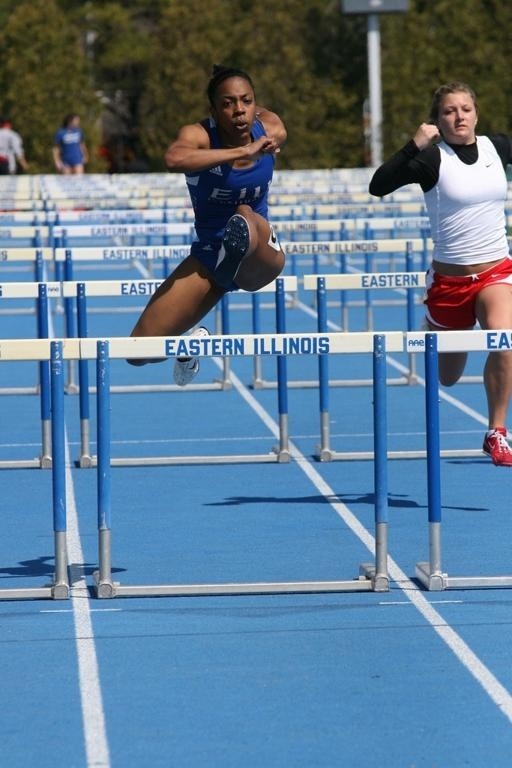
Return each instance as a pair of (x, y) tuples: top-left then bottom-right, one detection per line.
(172, 327), (211, 386)
(483, 428), (512, 465)
(212, 215), (251, 289)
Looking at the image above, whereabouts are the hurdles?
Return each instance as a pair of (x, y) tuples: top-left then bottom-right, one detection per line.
(0, 338), (79, 600)
(0, 168), (512, 470)
(80, 331), (404, 599)
(407, 330), (512, 592)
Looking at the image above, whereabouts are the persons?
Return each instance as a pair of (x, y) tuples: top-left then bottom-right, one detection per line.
(0, 122), (30, 175)
(52, 113), (89, 175)
(125, 64), (287, 387)
(368, 82), (512, 467)
